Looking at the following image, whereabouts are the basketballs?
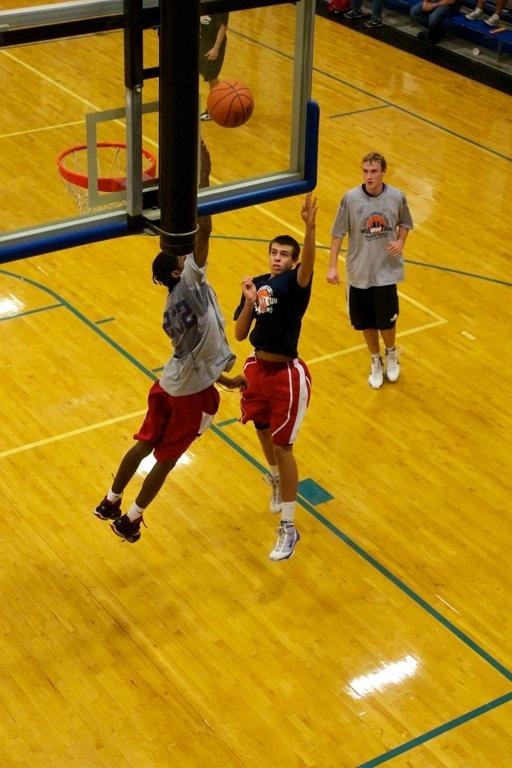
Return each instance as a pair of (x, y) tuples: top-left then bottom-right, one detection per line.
(207, 81), (254, 128)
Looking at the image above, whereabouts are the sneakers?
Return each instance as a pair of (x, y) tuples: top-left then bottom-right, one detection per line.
(93, 495), (123, 521)
(344, 11), (362, 19)
(265, 473), (282, 514)
(484, 14), (500, 27)
(418, 31), (433, 41)
(200, 112), (212, 121)
(269, 523), (300, 561)
(368, 357), (383, 389)
(364, 18), (383, 29)
(464, 9), (484, 21)
(385, 348), (399, 382)
(110, 513), (147, 543)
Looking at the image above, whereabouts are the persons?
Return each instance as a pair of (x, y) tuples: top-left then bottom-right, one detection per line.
(92, 132), (247, 543)
(344, 0), (384, 29)
(326, 152), (413, 389)
(411, 0), (463, 41)
(465, 0), (508, 28)
(234, 191), (318, 560)
(200, 11), (229, 123)
